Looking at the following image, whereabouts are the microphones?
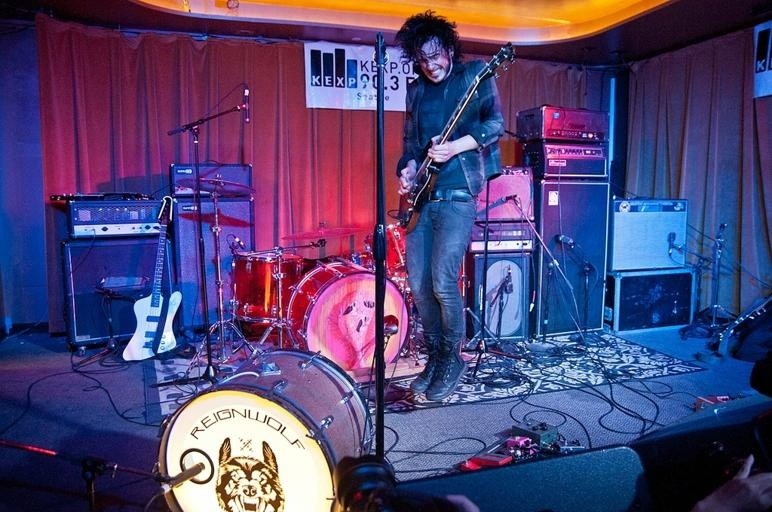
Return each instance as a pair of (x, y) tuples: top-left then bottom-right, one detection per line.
(114, 289), (149, 304)
(374, 32), (389, 64)
(156, 463), (205, 498)
(667, 231), (676, 257)
(506, 268), (513, 294)
(384, 316), (399, 351)
(242, 87), (250, 124)
(231, 233), (246, 250)
(554, 233), (575, 247)
(502, 192), (517, 204)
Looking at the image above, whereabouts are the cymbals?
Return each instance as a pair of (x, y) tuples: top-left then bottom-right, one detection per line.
(283, 228), (366, 240)
(199, 178), (256, 194)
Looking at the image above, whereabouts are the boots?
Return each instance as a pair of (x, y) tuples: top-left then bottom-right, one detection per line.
(410, 333), (467, 401)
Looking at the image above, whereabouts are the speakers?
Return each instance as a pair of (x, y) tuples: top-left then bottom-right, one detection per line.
(174, 195), (252, 331)
(471, 251), (531, 342)
(397, 444), (646, 512)
(64, 240), (175, 345)
(535, 178), (609, 343)
(607, 196), (689, 272)
(626, 395), (772, 512)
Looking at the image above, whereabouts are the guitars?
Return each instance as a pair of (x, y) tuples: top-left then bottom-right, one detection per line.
(120, 196), (182, 364)
(399, 43), (517, 235)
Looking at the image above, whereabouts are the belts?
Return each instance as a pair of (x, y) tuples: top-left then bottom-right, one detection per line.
(426, 188), (470, 202)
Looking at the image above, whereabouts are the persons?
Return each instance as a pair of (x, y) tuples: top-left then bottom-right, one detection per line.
(394, 9), (505, 400)
(447, 495), (480, 512)
(693, 454), (772, 512)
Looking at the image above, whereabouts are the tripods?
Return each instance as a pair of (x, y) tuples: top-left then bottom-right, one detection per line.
(185, 195), (253, 377)
(680, 243), (741, 343)
(151, 135), (225, 389)
(249, 257), (302, 352)
(461, 143), (520, 377)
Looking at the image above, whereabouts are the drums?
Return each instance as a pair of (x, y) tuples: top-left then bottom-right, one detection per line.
(363, 222), (406, 274)
(231, 248), (412, 372)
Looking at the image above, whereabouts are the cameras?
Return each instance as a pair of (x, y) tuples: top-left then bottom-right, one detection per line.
(331, 453), (463, 512)
(704, 438), (761, 489)
